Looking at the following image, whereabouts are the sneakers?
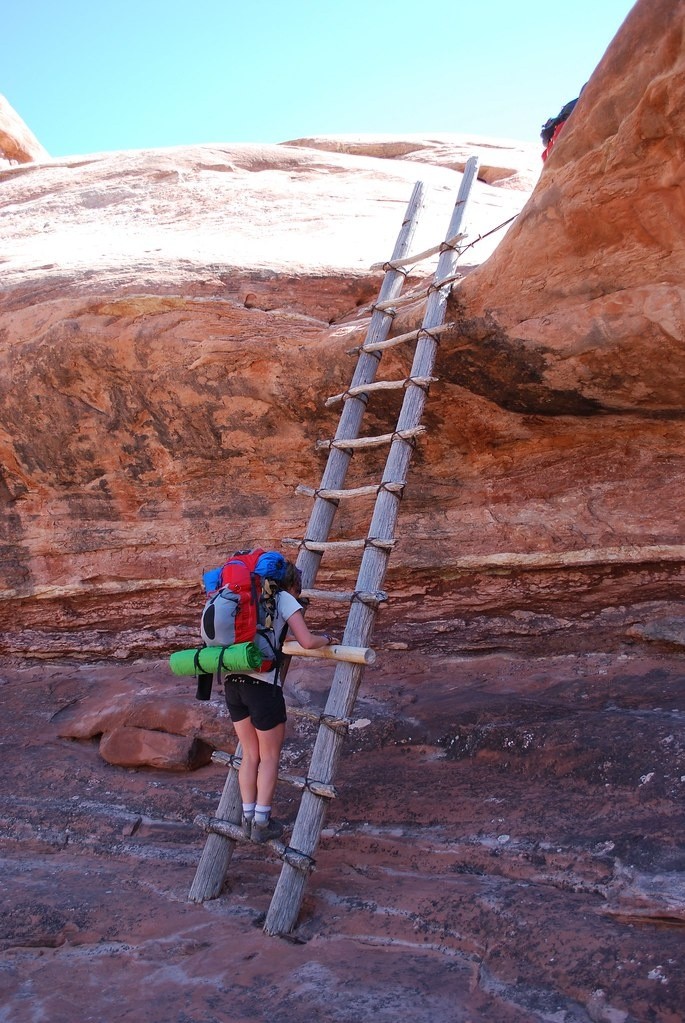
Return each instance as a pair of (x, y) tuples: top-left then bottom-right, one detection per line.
(241, 813), (253, 836)
(250, 813), (283, 844)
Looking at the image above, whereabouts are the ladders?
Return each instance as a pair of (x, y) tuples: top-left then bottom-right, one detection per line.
(186, 154), (481, 937)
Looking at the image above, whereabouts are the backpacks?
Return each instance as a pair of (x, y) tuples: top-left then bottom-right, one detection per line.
(200, 550), (293, 647)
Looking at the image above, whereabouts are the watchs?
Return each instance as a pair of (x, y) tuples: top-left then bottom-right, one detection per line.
(324, 634), (332, 644)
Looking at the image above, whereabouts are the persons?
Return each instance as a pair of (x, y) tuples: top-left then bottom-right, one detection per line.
(224, 561), (340, 843)
(541, 82), (587, 162)
